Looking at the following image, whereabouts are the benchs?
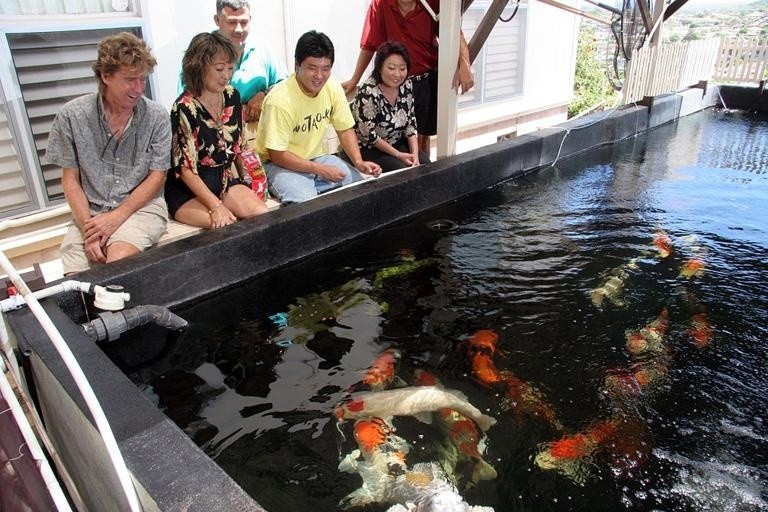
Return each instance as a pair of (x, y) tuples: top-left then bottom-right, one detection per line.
(146, 165), (385, 256)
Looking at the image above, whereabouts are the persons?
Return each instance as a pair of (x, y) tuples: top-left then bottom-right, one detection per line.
(43, 31), (171, 276)
(164, 31), (270, 232)
(253, 30), (384, 206)
(341, 0), (474, 159)
(177, 0), (286, 150)
(339, 41), (432, 172)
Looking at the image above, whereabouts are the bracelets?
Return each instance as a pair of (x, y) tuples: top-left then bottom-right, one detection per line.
(199, 91), (223, 127)
(395, 150), (399, 159)
(207, 199), (222, 213)
